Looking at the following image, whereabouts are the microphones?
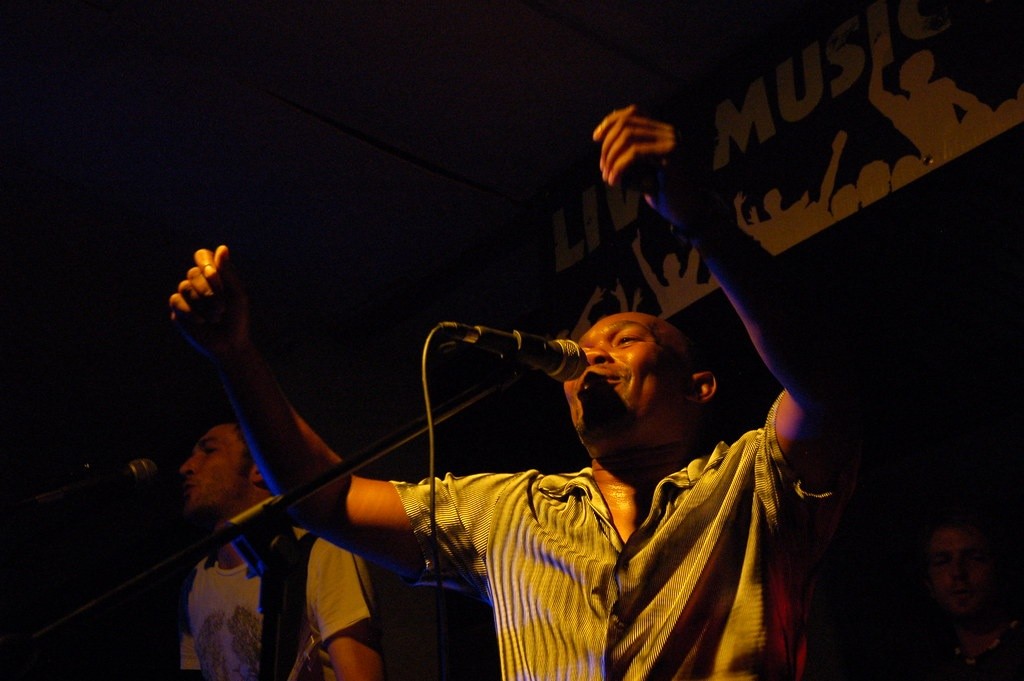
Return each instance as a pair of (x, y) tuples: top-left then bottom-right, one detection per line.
(73, 458), (158, 487)
(439, 322), (588, 382)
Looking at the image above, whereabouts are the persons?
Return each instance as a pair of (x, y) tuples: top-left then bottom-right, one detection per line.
(913, 503), (1024, 681)
(166, 106), (862, 681)
(176, 414), (386, 681)
